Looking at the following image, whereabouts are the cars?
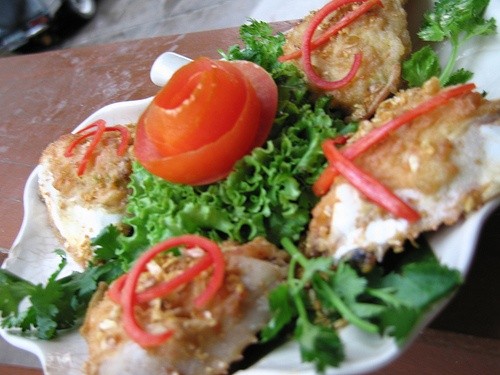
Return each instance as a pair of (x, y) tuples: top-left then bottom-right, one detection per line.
(0, 0), (96, 56)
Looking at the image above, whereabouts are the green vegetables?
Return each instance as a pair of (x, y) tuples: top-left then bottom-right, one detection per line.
(0, 0), (500, 375)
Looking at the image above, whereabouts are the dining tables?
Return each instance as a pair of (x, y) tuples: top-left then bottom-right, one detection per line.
(0, 17), (500, 375)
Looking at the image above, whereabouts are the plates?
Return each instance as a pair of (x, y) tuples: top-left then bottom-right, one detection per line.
(0, 0), (499, 374)
